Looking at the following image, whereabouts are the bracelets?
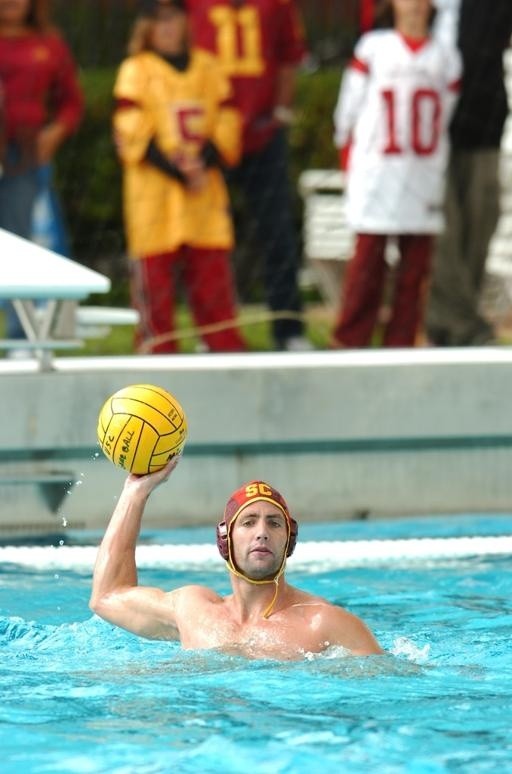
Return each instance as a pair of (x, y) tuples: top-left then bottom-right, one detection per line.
(274, 106), (295, 125)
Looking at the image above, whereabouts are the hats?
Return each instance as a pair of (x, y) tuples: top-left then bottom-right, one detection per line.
(217, 481), (298, 576)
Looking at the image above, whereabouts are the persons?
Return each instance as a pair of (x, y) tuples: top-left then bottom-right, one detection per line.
(90, 456), (423, 675)
(328, 0), (464, 348)
(371, 0), (512, 348)
(186, 0), (317, 353)
(113, 0), (246, 354)
(0, 0), (85, 359)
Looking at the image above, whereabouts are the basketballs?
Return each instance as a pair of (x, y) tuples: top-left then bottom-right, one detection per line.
(97, 383), (187, 475)
